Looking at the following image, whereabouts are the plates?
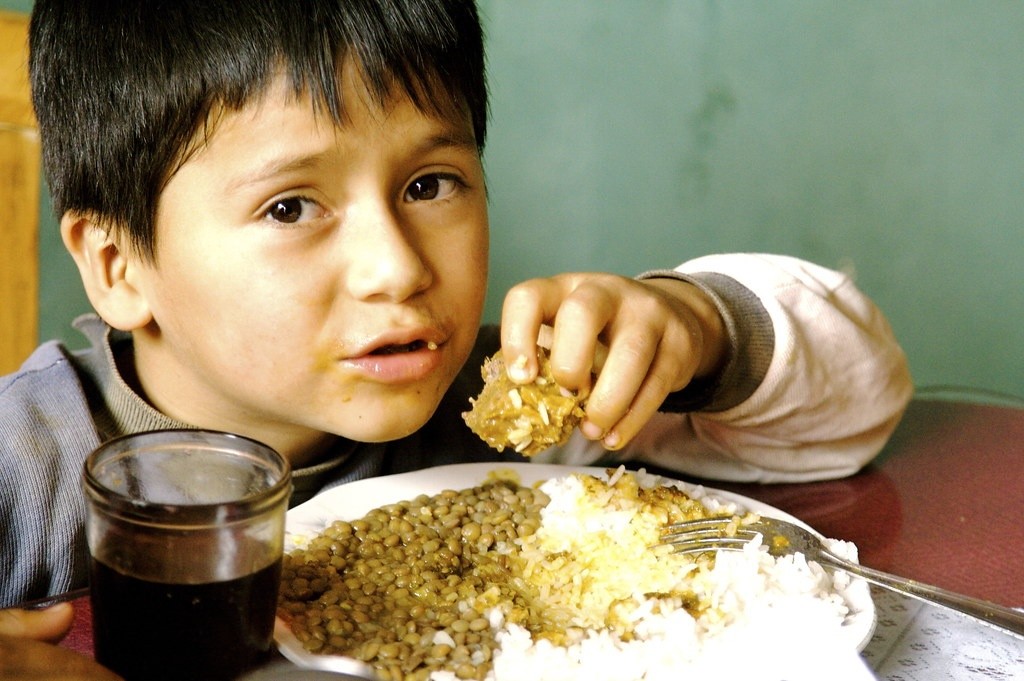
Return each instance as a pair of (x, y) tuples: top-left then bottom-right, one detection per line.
(274, 463), (876, 681)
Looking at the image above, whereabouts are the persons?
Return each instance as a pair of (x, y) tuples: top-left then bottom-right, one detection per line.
(1, 2), (915, 681)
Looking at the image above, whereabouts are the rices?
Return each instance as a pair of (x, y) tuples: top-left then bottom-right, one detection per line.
(427, 390), (864, 681)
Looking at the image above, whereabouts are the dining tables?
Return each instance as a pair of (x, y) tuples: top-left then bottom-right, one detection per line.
(38, 396), (1024, 681)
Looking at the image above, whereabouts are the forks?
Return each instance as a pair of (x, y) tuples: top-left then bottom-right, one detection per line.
(659, 515), (1024, 637)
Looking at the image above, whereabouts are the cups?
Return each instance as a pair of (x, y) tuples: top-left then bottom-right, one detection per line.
(80, 427), (293, 681)
